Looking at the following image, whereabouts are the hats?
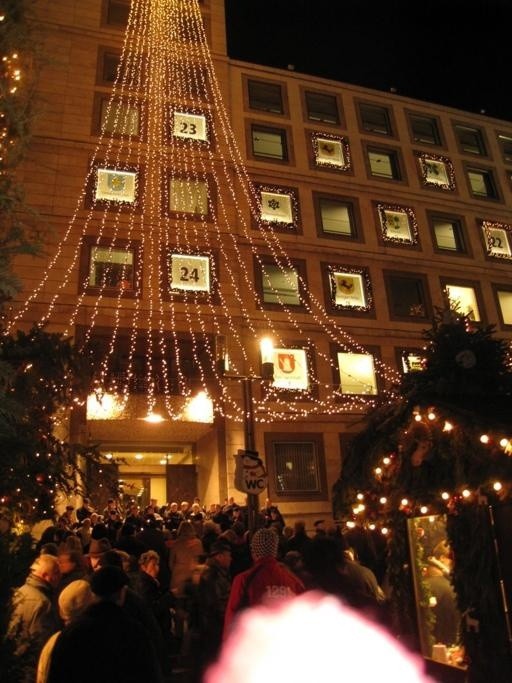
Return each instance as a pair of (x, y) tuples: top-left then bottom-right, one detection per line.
(83, 538), (116, 558)
(252, 528), (279, 558)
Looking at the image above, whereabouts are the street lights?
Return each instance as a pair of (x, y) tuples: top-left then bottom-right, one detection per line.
(213, 334), (277, 543)
